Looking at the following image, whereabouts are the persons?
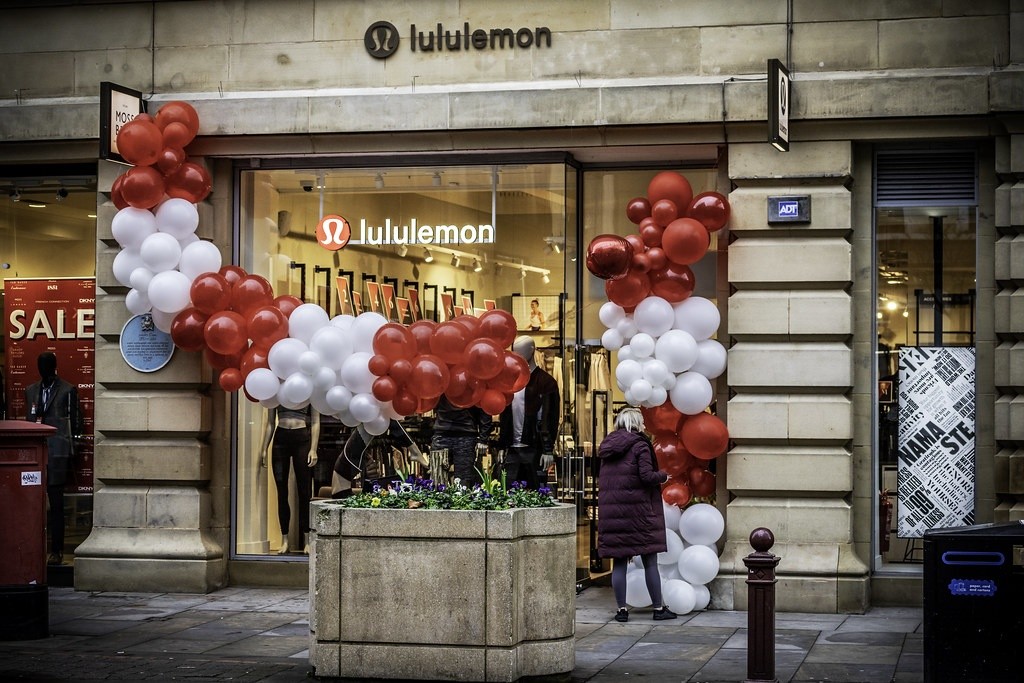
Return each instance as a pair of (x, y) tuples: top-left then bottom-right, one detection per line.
(429, 394), (492, 490)
(597, 408), (677, 621)
(500, 336), (561, 494)
(261, 404), (320, 555)
(332, 418), (428, 498)
(527, 300), (544, 330)
(25, 353), (80, 564)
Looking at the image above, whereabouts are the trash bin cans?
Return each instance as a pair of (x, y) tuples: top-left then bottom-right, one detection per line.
(921, 519), (1024, 683)
(0, 420), (57, 640)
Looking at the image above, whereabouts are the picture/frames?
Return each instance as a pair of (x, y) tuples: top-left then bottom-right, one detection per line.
(878, 381), (893, 404)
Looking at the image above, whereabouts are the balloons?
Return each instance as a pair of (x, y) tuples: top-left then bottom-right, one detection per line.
(587, 172), (730, 615)
(111, 102), (529, 434)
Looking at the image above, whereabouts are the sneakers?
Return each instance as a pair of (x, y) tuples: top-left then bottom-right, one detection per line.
(615, 609), (629, 622)
(653, 605), (677, 620)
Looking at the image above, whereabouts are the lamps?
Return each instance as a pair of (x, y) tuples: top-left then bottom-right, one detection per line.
(396, 243), (408, 257)
(56, 188), (68, 202)
(423, 247), (433, 262)
(316, 174), (326, 188)
(902, 307), (909, 318)
(449, 182), (459, 187)
(279, 210), (293, 236)
(494, 263), (503, 277)
(374, 171), (384, 188)
(432, 171), (441, 187)
(542, 244), (554, 255)
(540, 273), (551, 285)
(29, 204), (47, 208)
(516, 269), (526, 281)
(472, 258), (483, 273)
(492, 173), (500, 184)
(451, 253), (461, 268)
(8, 186), (22, 203)
(555, 243), (564, 254)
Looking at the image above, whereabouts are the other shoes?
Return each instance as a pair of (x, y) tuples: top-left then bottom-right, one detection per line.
(47, 553), (63, 564)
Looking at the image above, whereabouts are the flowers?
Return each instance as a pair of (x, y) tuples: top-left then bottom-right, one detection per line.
(337, 464), (559, 511)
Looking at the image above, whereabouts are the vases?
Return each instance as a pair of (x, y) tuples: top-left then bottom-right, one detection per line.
(308, 498), (577, 683)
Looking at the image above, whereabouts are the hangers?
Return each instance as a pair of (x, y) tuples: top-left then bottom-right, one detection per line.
(591, 346), (606, 358)
